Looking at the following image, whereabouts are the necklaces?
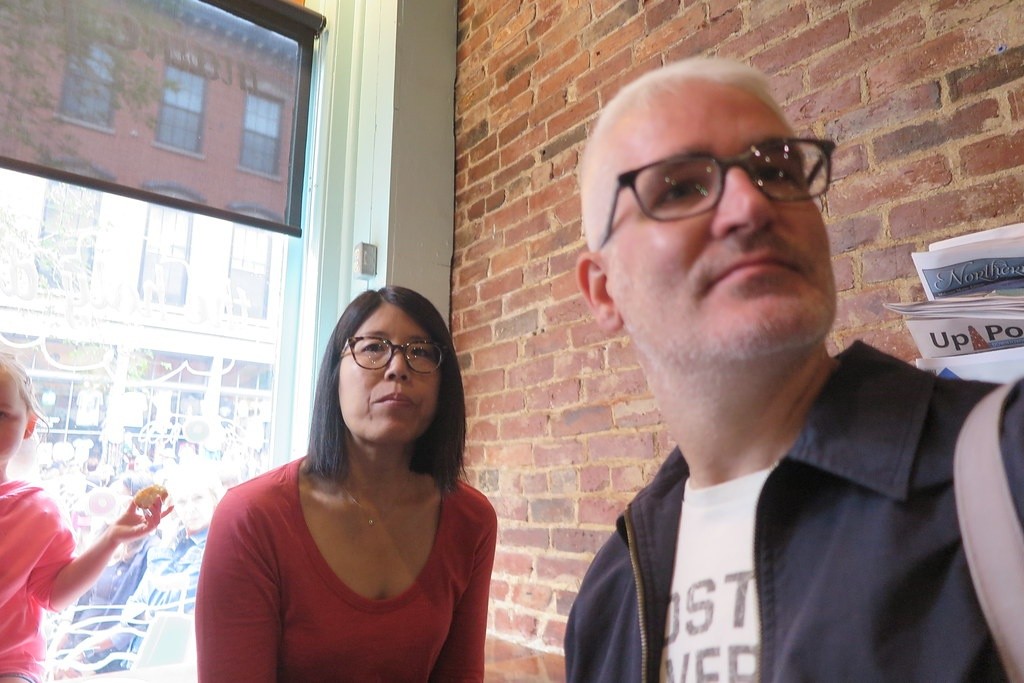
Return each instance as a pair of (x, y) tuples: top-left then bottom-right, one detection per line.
(342, 476), (411, 527)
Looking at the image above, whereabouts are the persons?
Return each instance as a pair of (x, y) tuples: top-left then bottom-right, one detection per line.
(195, 286), (498, 683)
(561, 57), (1024, 683)
(0, 358), (214, 683)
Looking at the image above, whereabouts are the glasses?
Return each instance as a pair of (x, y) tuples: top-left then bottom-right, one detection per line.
(595, 137), (837, 253)
(338, 336), (443, 375)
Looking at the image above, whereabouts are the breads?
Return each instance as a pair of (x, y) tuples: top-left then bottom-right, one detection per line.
(133, 483), (167, 508)
